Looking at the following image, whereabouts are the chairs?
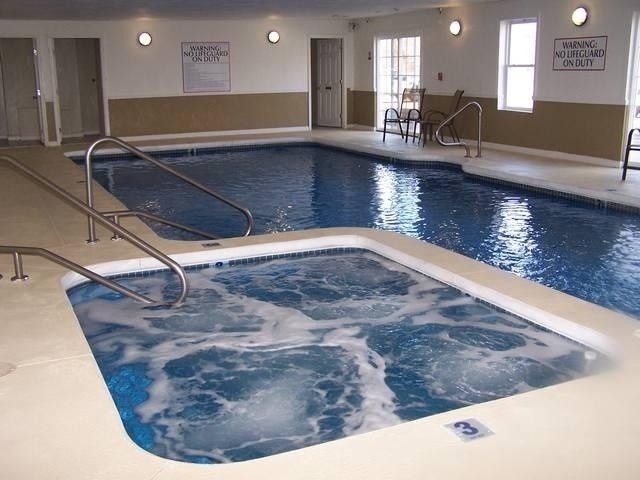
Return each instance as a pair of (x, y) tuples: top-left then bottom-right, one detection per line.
(412, 89), (464, 147)
(382, 88), (426, 143)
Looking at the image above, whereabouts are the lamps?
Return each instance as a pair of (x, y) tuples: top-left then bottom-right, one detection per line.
(449, 21), (461, 35)
(268, 31), (280, 43)
(138, 32), (151, 46)
(572, 7), (587, 26)
